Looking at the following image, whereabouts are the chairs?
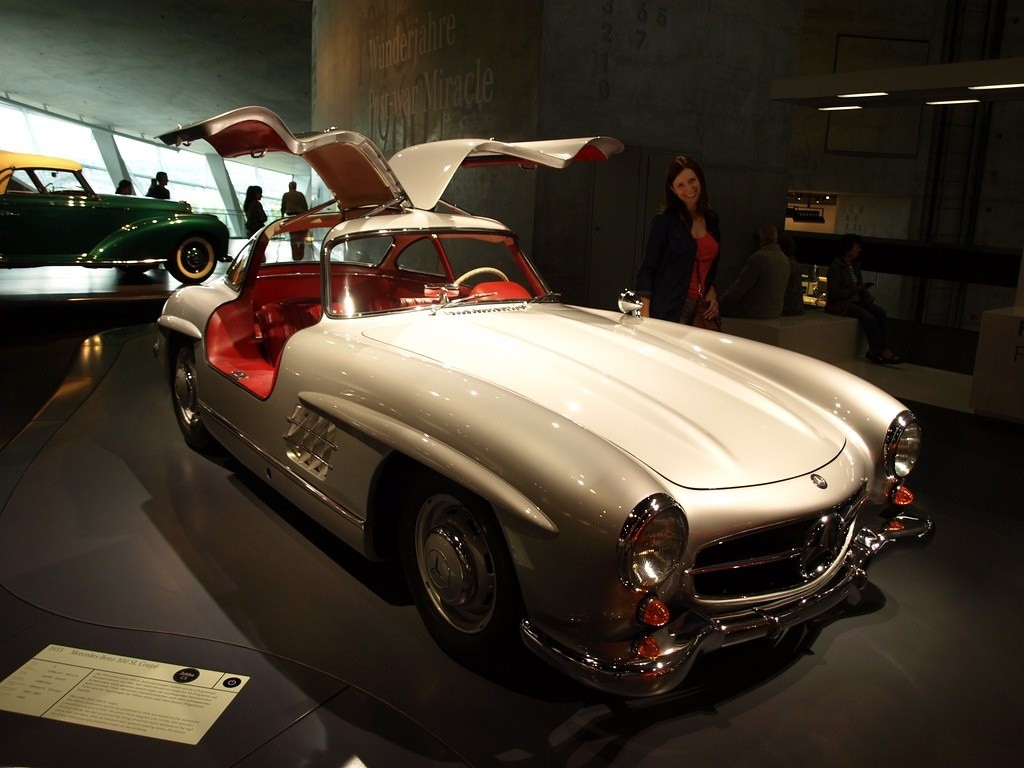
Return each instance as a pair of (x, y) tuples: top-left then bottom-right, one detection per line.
(256, 294), (349, 367)
(350, 295), (435, 315)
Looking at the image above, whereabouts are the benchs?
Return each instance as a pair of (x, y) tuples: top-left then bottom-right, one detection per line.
(717, 307), (876, 361)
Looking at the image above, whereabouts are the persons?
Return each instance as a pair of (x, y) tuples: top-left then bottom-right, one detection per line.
(633, 155), (723, 332)
(825, 234), (902, 363)
(242, 185), (267, 239)
(145, 172), (170, 199)
(280, 182), (308, 261)
(726, 226), (788, 319)
(115, 178), (132, 193)
(780, 238), (804, 317)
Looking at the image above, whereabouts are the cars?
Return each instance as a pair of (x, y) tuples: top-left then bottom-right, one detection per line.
(147, 102), (932, 710)
(0, 155), (234, 286)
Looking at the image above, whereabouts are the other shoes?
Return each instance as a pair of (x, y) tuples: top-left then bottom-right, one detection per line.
(865, 348), (904, 365)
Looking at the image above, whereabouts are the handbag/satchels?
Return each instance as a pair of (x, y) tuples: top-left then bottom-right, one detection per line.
(691, 297), (722, 332)
